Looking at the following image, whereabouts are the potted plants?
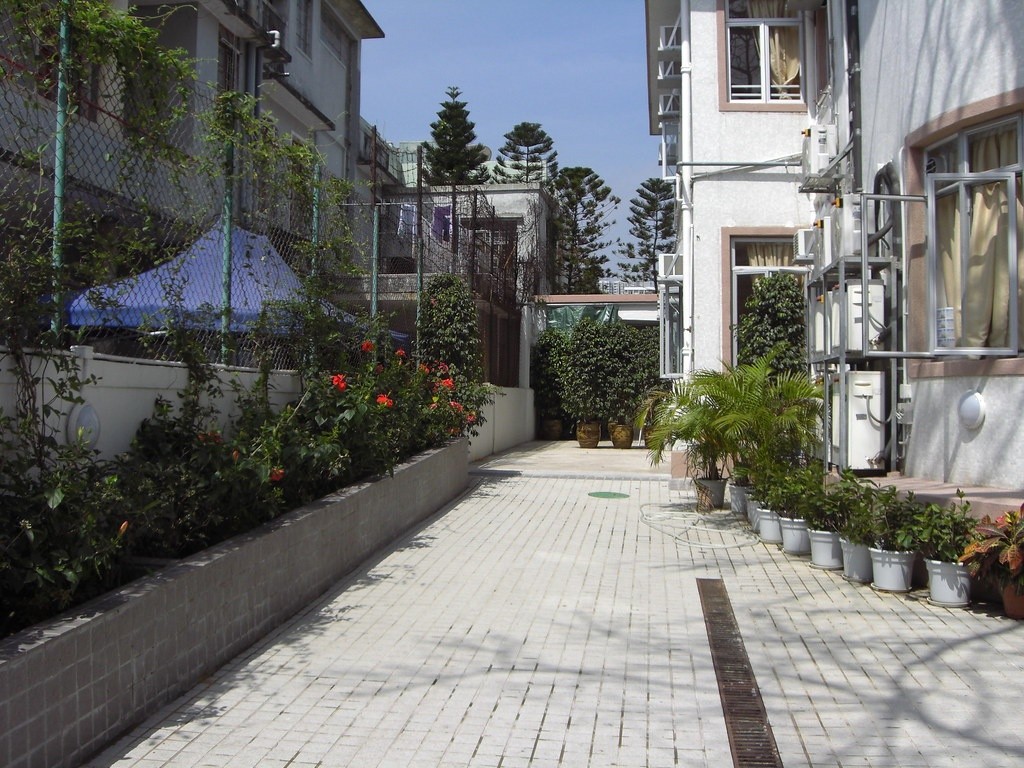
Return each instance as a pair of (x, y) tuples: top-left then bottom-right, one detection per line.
(631, 341), (1024, 619)
(531, 317), (672, 449)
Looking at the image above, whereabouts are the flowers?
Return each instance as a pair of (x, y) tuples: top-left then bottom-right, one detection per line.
(0, 0), (506, 638)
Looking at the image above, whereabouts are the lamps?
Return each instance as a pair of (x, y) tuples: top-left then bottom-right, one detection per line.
(67, 346), (101, 453)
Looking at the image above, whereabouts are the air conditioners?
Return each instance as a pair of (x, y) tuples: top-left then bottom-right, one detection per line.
(659, 254), (682, 278)
(794, 229), (815, 258)
(815, 279), (885, 351)
(814, 217), (831, 276)
(801, 125), (837, 188)
(831, 195), (893, 261)
(806, 371), (886, 469)
